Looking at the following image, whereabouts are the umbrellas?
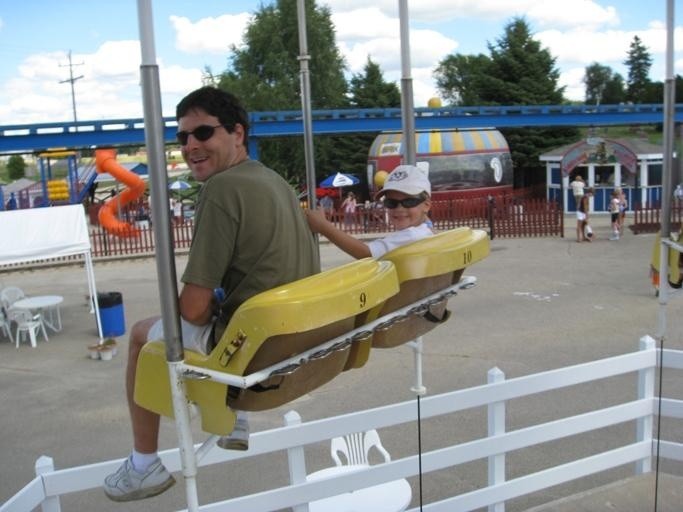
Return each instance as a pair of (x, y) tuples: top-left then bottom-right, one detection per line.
(313, 187), (339, 199)
(167, 181), (191, 203)
(319, 171), (360, 204)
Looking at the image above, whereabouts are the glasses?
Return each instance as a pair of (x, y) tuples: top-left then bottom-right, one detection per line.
(176, 125), (223, 146)
(384, 198), (424, 209)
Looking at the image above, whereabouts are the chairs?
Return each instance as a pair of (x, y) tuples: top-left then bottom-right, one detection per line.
(0, 284), (65, 351)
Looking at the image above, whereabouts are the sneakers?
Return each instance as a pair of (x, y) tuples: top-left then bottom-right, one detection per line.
(217, 420), (248, 450)
(104, 455), (176, 501)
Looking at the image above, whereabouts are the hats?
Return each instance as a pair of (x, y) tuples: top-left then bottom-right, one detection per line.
(373, 165), (431, 201)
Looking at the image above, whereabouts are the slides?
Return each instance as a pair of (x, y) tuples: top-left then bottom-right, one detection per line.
(95, 150), (145, 237)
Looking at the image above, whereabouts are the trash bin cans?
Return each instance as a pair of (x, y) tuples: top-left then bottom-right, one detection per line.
(92, 292), (126, 338)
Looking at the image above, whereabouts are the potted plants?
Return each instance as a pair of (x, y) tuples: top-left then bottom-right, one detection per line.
(85, 332), (118, 362)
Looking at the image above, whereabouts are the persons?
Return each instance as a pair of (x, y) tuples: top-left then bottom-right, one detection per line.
(583, 221), (592, 242)
(87, 181), (96, 206)
(103, 87), (321, 500)
(616, 192), (626, 237)
(8, 193), (17, 210)
(305, 165), (435, 260)
(110, 188), (115, 197)
(340, 192), (357, 233)
(570, 176), (585, 216)
(575, 188), (594, 243)
(319, 193), (333, 221)
(606, 190), (620, 241)
(172, 199), (185, 227)
